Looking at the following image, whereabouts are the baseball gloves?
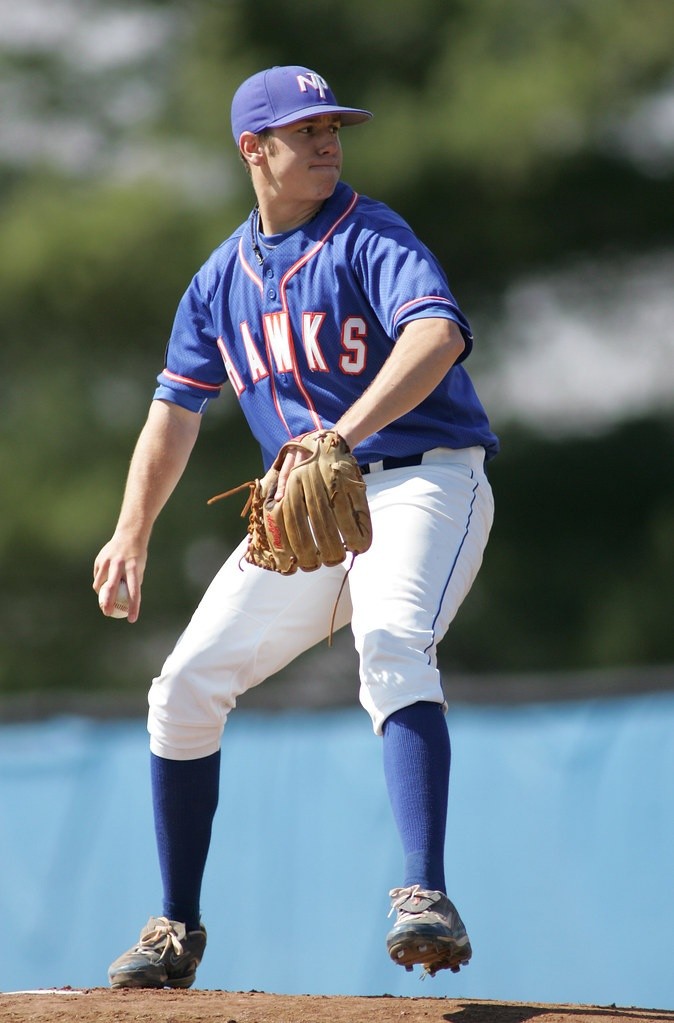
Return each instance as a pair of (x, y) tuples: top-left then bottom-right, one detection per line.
(204, 427), (374, 578)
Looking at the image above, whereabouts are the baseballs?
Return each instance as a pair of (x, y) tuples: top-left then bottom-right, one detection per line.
(97, 578), (131, 620)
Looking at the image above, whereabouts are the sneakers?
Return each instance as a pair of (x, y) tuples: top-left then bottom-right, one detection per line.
(386, 884), (472, 977)
(107, 916), (207, 989)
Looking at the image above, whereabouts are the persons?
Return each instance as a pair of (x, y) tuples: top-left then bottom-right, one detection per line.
(91, 64), (498, 988)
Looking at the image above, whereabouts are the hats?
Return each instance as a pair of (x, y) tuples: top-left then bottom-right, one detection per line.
(230, 65), (374, 147)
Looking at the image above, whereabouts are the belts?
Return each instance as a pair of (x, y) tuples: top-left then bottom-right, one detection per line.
(359, 451), (424, 474)
